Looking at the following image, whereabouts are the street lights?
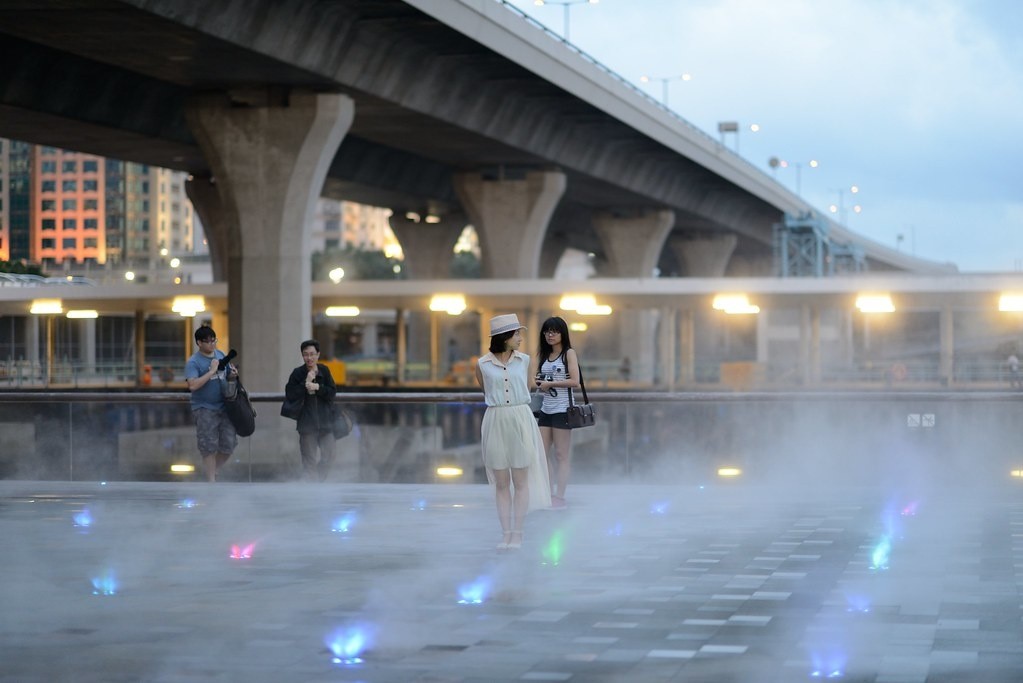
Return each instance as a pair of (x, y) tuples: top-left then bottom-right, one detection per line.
(779, 158), (819, 197)
(829, 186), (861, 228)
(537, 0), (597, 42)
(640, 71), (692, 109)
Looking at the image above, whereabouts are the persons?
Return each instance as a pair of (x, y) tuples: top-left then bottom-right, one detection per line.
(475, 313), (552, 554)
(288, 340), (338, 483)
(185, 326), (238, 484)
(1007, 349), (1022, 388)
(533, 316), (579, 507)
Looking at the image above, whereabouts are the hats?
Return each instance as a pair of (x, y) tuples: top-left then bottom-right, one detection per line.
(489, 314), (527, 337)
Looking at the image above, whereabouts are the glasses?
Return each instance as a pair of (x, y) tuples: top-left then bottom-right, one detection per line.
(543, 330), (562, 337)
(302, 352), (320, 357)
(201, 339), (218, 345)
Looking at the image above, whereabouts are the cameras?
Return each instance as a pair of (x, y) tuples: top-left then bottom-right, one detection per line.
(537, 374), (552, 387)
(217, 350), (238, 371)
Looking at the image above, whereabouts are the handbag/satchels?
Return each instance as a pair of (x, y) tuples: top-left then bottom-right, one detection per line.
(567, 403), (597, 428)
(332, 404), (353, 440)
(280, 397), (304, 420)
(225, 377), (255, 436)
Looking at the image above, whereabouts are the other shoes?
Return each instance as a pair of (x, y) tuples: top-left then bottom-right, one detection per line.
(544, 494), (567, 511)
(498, 529), (522, 550)
